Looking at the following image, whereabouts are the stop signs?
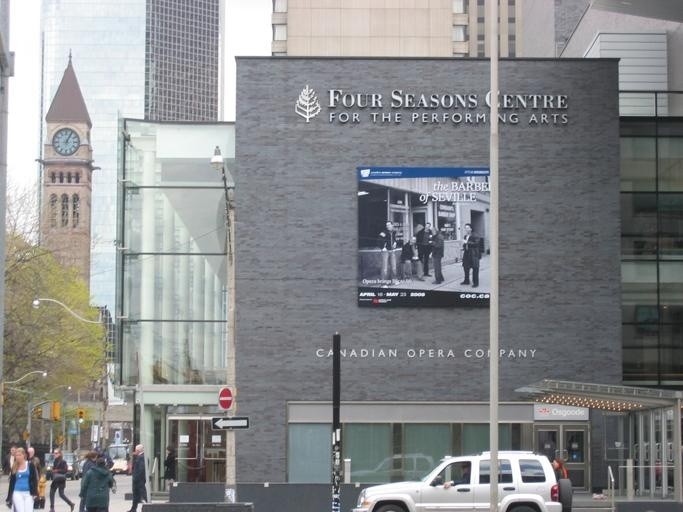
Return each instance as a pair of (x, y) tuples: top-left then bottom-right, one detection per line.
(218, 388), (233, 410)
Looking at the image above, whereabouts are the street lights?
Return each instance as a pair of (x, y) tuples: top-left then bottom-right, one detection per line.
(0, 369), (72, 475)
(30, 296), (107, 326)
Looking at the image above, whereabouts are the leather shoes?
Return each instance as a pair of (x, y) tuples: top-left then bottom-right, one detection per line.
(71, 504), (74, 512)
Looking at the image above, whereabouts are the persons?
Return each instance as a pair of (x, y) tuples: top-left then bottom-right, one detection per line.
(163, 443), (176, 492)
(374, 220), (399, 288)
(551, 456), (564, 481)
(458, 222), (480, 288)
(399, 235), (425, 283)
(416, 221), (432, 277)
(428, 226), (444, 285)
(125, 443), (146, 511)
(441, 461), (470, 489)
(1, 445), (115, 511)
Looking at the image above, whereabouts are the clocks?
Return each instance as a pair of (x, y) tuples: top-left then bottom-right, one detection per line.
(51, 128), (81, 156)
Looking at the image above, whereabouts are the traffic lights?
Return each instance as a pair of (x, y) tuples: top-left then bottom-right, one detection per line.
(76, 409), (85, 424)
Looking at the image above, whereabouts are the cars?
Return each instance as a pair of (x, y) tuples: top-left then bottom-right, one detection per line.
(44, 440), (134, 482)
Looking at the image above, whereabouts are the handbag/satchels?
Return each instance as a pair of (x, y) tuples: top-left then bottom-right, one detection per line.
(53, 473), (65, 482)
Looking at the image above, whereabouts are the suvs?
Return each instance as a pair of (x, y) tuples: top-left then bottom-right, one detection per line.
(350, 448), (573, 512)
(348, 452), (435, 484)
